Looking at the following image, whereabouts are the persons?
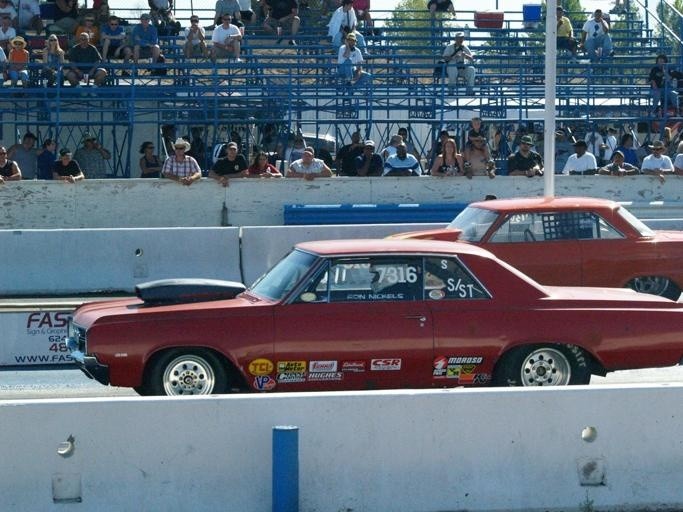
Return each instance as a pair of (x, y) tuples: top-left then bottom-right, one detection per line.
(368, 261), (412, 299)
(428, 0), (476, 95)
(555, 5), (682, 185)
(138, 117), (546, 187)
(1, 132), (111, 183)
(0, 0), (375, 81)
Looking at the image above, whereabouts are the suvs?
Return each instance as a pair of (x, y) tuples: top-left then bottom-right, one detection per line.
(263, 131), (340, 168)
(213, 142), (262, 170)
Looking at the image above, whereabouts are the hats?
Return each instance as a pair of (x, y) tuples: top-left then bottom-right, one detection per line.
(346, 33), (357, 42)
(304, 147), (314, 156)
(24, 133), (38, 141)
(228, 142), (237, 150)
(520, 135), (533, 147)
(171, 138), (190, 152)
(648, 140), (667, 154)
(365, 140), (375, 147)
(456, 32), (464, 38)
(81, 132), (96, 143)
(571, 140), (587, 146)
(12, 36), (26, 48)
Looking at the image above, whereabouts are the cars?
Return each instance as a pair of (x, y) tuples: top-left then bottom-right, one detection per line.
(381, 195), (683, 305)
(59, 240), (683, 396)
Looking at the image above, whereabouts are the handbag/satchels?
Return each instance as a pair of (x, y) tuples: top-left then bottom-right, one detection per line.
(436, 60), (446, 72)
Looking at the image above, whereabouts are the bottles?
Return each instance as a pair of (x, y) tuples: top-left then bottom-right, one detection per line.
(672, 78), (678, 88)
(597, 47), (602, 56)
(463, 55), (468, 65)
(266, 166), (271, 176)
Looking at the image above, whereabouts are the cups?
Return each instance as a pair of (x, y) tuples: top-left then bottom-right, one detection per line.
(89, 32), (94, 38)
(239, 27), (244, 35)
(83, 73), (88, 83)
(41, 20), (47, 27)
(618, 168), (624, 176)
(120, 32), (125, 40)
(276, 27), (282, 34)
